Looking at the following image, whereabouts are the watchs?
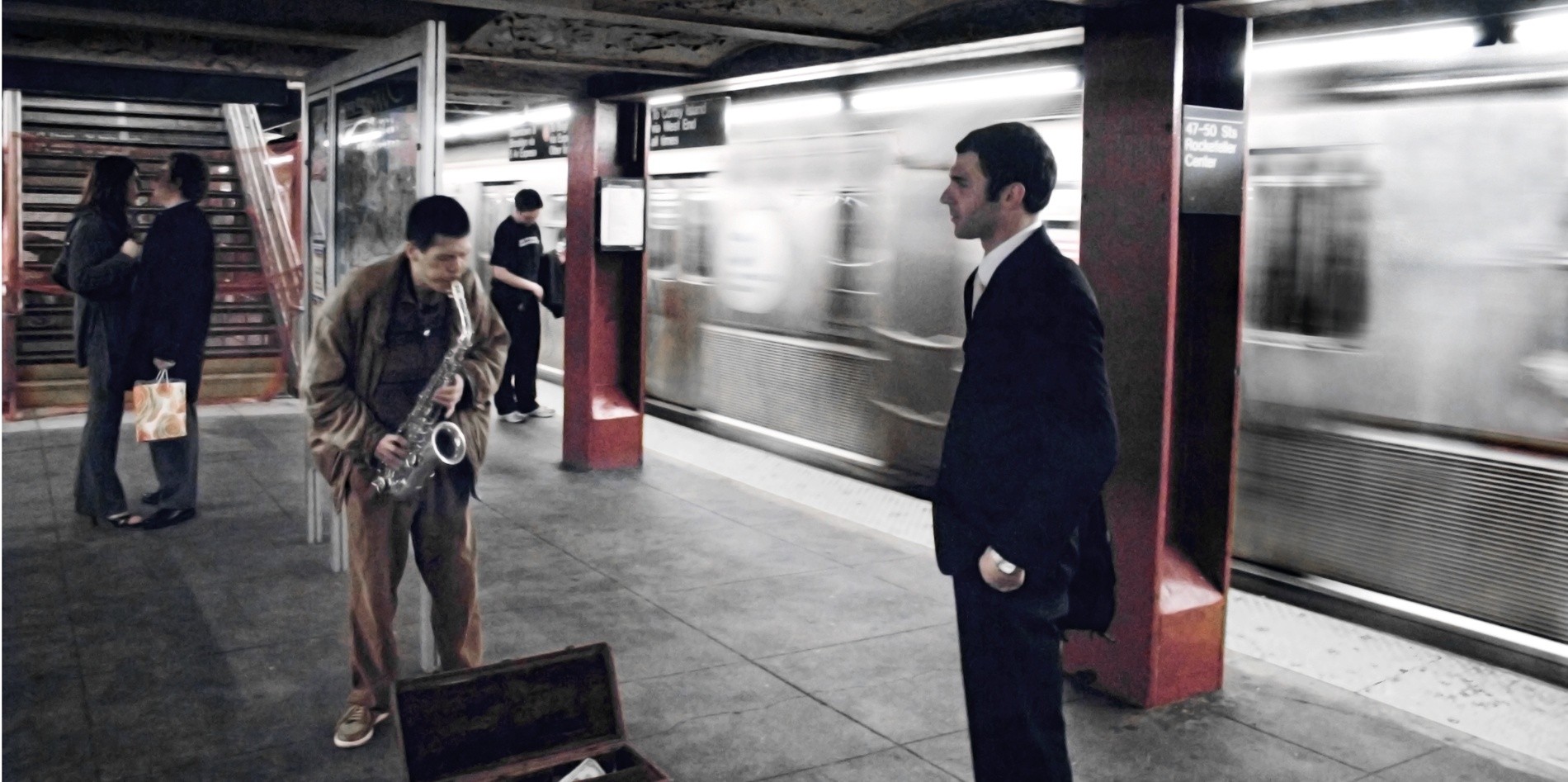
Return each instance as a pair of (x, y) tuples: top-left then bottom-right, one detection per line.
(988, 545), (1023, 577)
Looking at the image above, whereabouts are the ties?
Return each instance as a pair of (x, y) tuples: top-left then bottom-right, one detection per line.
(971, 271), (985, 317)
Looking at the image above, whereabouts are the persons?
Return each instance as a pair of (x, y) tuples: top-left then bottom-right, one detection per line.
(65, 152), (215, 531)
(487, 189), (566, 423)
(930, 122), (1120, 782)
(298, 196), (512, 749)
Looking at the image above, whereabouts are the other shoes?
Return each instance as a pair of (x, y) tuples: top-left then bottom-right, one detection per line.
(526, 404), (554, 416)
(497, 411), (528, 423)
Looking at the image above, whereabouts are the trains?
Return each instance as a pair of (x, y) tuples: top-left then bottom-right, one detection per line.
(445, 0), (1568, 690)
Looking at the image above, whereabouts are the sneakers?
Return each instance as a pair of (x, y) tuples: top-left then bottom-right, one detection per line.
(334, 705), (391, 748)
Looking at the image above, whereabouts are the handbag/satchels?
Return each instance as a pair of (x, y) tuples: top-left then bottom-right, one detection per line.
(132, 368), (188, 441)
(52, 214), (87, 288)
(1052, 493), (1118, 631)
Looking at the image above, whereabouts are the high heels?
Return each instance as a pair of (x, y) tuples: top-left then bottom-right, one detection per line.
(87, 509), (142, 529)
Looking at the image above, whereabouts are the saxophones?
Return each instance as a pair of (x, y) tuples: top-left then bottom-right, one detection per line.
(378, 279), (472, 508)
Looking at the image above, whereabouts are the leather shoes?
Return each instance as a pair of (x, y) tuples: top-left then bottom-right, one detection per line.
(141, 507), (195, 530)
(140, 489), (165, 504)
